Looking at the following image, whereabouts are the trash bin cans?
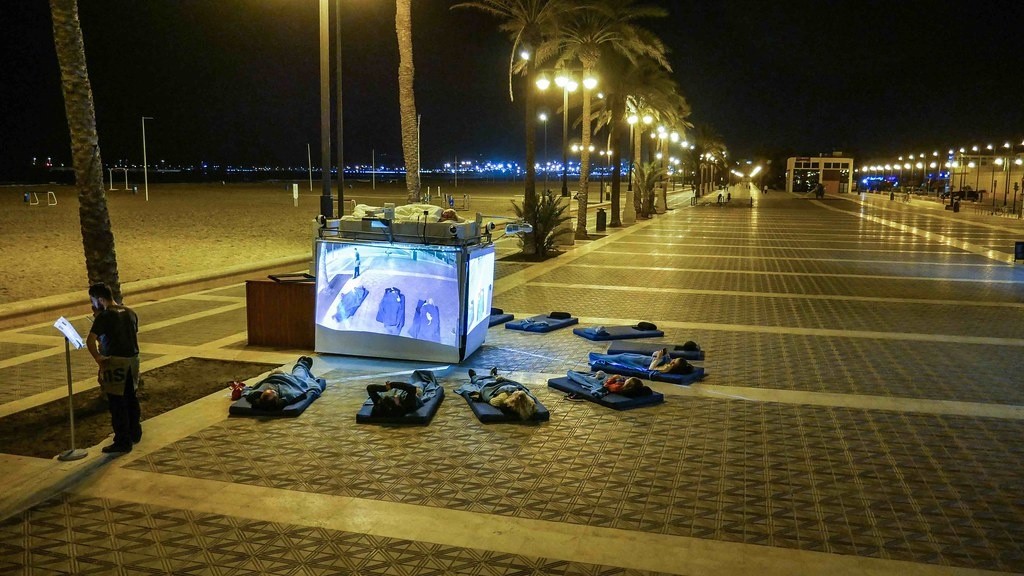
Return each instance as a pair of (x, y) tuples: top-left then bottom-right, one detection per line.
(596, 207), (607, 230)
(954, 201), (959, 212)
(890, 192), (894, 200)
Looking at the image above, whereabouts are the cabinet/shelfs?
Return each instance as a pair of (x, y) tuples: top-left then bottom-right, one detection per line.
(244, 278), (315, 349)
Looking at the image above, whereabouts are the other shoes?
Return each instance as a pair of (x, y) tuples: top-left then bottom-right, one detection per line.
(306, 357), (313, 369)
(102, 443), (132, 453)
(567, 394), (584, 402)
(469, 369), (476, 379)
(297, 356), (306, 364)
(563, 392), (575, 400)
(131, 438), (140, 442)
(490, 366), (497, 375)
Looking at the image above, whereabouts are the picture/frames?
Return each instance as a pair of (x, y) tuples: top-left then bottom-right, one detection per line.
(268, 273), (315, 282)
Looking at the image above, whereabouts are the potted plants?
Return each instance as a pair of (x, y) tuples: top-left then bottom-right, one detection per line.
(506, 188), (577, 256)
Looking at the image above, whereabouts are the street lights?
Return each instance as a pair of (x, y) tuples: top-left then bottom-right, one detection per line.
(898, 155), (904, 180)
(599, 150), (605, 203)
(972, 146), (981, 192)
(995, 158), (1008, 206)
(959, 147), (968, 187)
(658, 126), (665, 188)
(627, 116), (637, 191)
(1004, 142), (1014, 193)
(142, 116), (154, 201)
(908, 154), (915, 187)
(855, 157), (1023, 193)
(987, 143), (996, 193)
(919, 153), (926, 181)
(536, 66), (599, 197)
(933, 151), (940, 197)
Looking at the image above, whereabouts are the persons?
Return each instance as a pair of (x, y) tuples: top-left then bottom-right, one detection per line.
(566, 347), (694, 398)
(421, 193), (428, 203)
(364, 368), (440, 423)
(349, 199), (465, 223)
(574, 193), (579, 199)
(86, 283), (143, 452)
(857, 183), (861, 195)
(761, 184), (768, 194)
(468, 367), (536, 424)
(352, 248), (360, 279)
(692, 184), (695, 193)
(467, 284), (492, 329)
(384, 245), (410, 256)
(449, 194), (468, 210)
(245, 355), (315, 414)
(332, 284), (440, 343)
(547, 189), (555, 202)
(132, 184), (139, 194)
(24, 191), (29, 202)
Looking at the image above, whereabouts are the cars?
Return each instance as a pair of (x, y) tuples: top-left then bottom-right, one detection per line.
(953, 191), (979, 202)
(914, 188), (924, 195)
(940, 191), (953, 199)
